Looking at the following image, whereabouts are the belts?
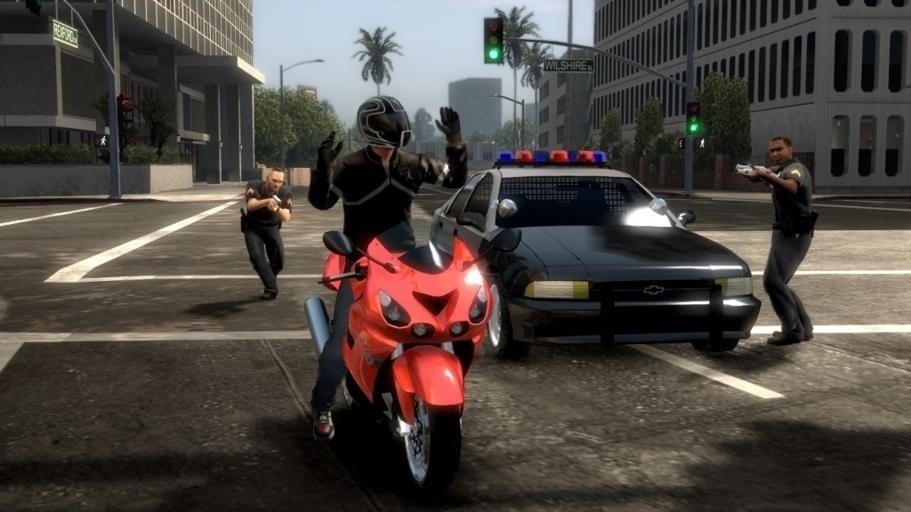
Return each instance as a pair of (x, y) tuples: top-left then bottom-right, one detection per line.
(773, 222), (800, 230)
(248, 222), (280, 227)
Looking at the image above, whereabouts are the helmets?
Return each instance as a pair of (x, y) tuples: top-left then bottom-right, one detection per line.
(357, 95), (412, 148)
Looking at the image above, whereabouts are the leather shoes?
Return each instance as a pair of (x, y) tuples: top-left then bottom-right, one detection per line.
(768, 331), (813, 346)
(261, 291), (276, 300)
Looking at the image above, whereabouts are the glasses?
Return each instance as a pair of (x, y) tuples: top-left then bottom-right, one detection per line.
(769, 147), (784, 152)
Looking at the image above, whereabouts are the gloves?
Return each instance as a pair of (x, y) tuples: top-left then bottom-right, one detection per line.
(316, 131), (343, 173)
(435, 106), (461, 143)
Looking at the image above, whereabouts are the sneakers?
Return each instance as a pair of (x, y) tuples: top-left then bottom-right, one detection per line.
(312, 410), (335, 441)
